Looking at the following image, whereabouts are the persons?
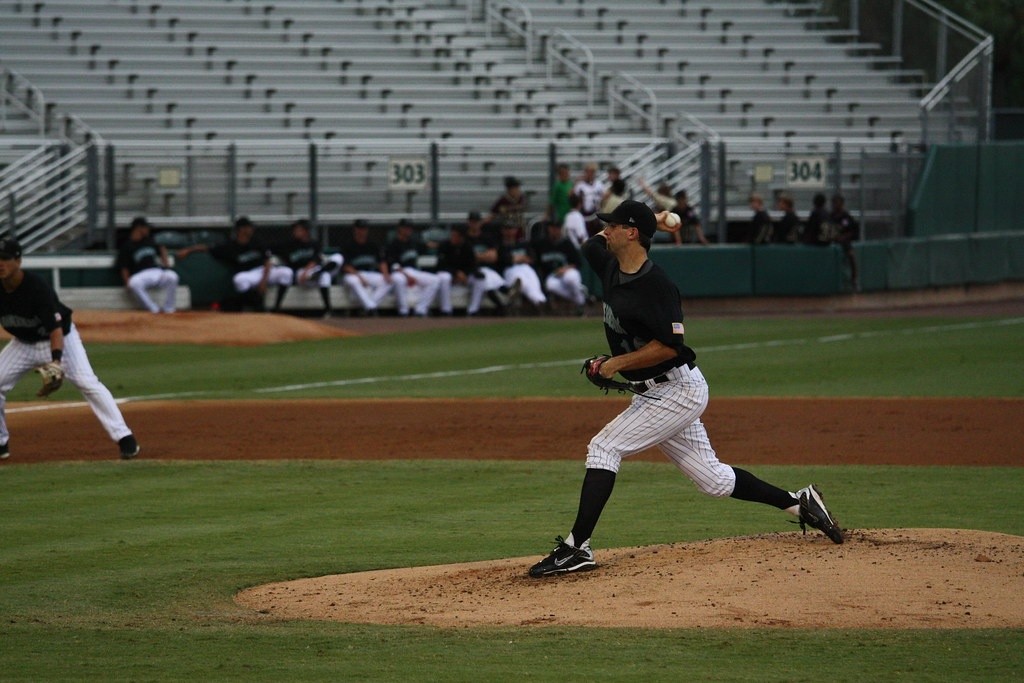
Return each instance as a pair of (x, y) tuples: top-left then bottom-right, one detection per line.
(0, 237), (140, 459)
(114, 159), (859, 314)
(526, 199), (844, 578)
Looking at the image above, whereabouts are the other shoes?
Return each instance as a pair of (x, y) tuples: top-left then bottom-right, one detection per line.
(570, 307), (584, 316)
(537, 301), (553, 316)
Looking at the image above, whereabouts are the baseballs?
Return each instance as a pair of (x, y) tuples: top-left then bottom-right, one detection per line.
(665, 212), (681, 228)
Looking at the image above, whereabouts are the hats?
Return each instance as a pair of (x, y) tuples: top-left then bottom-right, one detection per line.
(0, 234), (23, 260)
(595, 200), (658, 238)
(673, 190), (686, 200)
(828, 190), (847, 202)
(230, 212), (467, 236)
(607, 167), (621, 175)
(498, 220), (520, 230)
(776, 191), (794, 207)
(747, 193), (763, 202)
(130, 216), (151, 228)
(504, 177), (523, 189)
(546, 218), (564, 227)
(466, 209), (485, 220)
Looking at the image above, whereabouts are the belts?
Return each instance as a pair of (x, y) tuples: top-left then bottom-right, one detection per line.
(635, 361), (697, 393)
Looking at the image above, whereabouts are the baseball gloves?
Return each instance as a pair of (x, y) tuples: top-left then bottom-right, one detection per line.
(579, 352), (662, 401)
(34, 359), (66, 400)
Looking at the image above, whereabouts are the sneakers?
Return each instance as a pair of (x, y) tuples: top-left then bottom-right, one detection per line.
(786, 484), (847, 544)
(508, 278), (521, 299)
(529, 535), (598, 578)
(0, 442), (11, 460)
(118, 434), (141, 458)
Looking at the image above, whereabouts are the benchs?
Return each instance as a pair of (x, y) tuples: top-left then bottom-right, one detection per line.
(0, 0), (979, 215)
